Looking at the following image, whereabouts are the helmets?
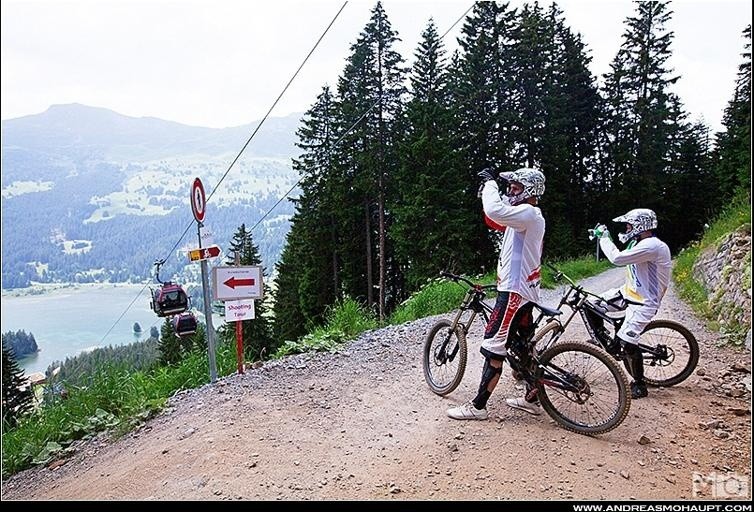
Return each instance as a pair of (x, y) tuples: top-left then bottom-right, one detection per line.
(499, 168), (545, 205)
(612, 209), (659, 242)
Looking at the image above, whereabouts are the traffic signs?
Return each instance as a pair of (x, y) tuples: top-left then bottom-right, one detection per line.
(188, 244), (223, 264)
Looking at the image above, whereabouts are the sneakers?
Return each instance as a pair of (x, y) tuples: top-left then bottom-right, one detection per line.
(448, 401), (487, 420)
(631, 381), (648, 399)
(507, 399), (542, 415)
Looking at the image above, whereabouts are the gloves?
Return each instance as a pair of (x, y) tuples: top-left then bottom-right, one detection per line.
(477, 167), (496, 197)
(589, 224), (612, 242)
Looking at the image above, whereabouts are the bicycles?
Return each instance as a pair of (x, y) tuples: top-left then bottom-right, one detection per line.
(422, 271), (632, 436)
(511, 262), (700, 388)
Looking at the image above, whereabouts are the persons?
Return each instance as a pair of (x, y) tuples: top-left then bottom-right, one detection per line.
(446, 166), (546, 420)
(581, 208), (673, 400)
